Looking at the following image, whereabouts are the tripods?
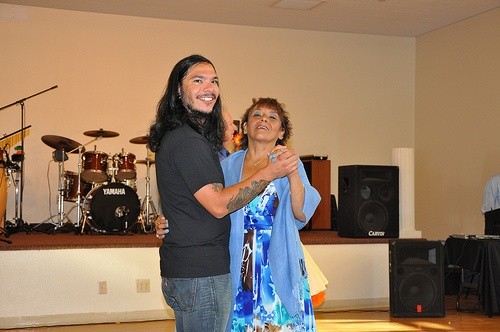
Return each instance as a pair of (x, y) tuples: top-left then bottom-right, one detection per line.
(27, 134), (101, 234)
(140, 145), (158, 229)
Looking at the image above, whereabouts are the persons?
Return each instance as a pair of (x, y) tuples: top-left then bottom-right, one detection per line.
(147, 55), (299, 332)
(156, 97), (322, 332)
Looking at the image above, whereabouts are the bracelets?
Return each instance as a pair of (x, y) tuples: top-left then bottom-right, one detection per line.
(289, 185), (305, 196)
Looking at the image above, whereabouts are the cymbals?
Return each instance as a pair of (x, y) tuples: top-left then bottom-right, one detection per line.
(41, 135), (86, 154)
(83, 129), (119, 138)
(136, 158), (156, 166)
(129, 134), (150, 144)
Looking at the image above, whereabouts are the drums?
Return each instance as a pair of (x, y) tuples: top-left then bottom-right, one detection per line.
(84, 183), (141, 234)
(0, 159), (8, 226)
(81, 150), (110, 182)
(112, 153), (137, 181)
(57, 171), (91, 203)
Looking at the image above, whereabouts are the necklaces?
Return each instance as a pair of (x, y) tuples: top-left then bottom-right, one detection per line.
(254, 161), (261, 166)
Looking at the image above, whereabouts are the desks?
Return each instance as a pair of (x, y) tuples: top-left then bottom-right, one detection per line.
(444, 238), (500, 318)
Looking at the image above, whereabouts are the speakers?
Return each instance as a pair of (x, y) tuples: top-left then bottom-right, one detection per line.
(390, 240), (445, 318)
(301, 159), (331, 230)
(338, 165), (399, 239)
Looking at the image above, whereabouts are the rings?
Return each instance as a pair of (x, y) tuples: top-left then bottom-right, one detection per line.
(158, 222), (160, 225)
